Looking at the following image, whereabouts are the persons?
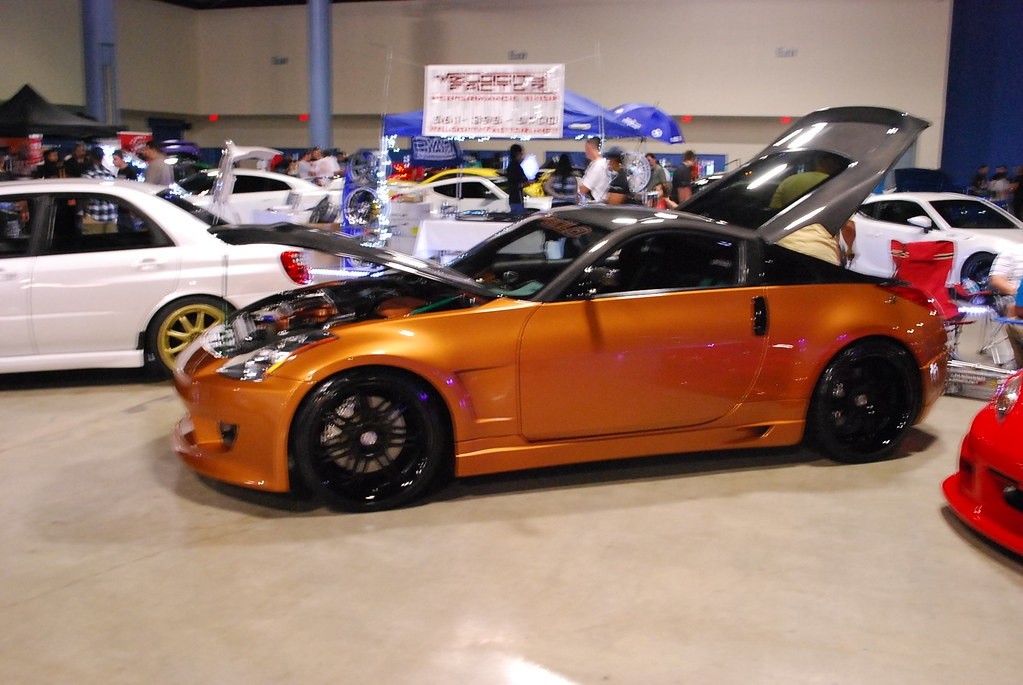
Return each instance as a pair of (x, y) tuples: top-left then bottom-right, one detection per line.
(769, 157), (856, 267)
(1005, 277), (1023, 369)
(112, 150), (137, 181)
(506, 144), (530, 222)
(31, 143), (88, 234)
(989, 244), (1023, 317)
(642, 153), (666, 192)
(545, 154), (578, 208)
(578, 137), (612, 204)
(648, 182), (673, 209)
(669, 150), (695, 205)
(0, 151), (29, 238)
(274, 147), (347, 187)
(80, 147), (118, 234)
(606, 153), (629, 204)
(972, 164), (1023, 222)
(144, 140), (174, 186)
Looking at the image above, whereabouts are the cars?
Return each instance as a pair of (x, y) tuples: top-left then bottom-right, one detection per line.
(836, 191), (1023, 309)
(388, 166), (562, 224)
(136, 162), (349, 228)
(0, 174), (351, 383)
(940, 365), (1023, 560)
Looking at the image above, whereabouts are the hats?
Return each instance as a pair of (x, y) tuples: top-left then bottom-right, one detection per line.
(601, 146), (625, 159)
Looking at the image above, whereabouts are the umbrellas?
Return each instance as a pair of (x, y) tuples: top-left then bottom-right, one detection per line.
(609, 100), (686, 152)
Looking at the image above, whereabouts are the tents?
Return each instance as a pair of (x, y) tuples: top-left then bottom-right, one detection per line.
(381, 89), (641, 177)
(0, 85), (129, 155)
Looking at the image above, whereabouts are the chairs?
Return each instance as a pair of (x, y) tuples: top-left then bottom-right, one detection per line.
(980, 278), (1023, 367)
(891, 238), (968, 361)
(636, 248), (692, 287)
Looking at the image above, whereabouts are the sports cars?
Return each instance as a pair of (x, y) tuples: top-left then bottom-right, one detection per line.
(169, 106), (949, 511)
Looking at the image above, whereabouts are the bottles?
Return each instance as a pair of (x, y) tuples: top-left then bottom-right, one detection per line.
(962, 276), (984, 303)
